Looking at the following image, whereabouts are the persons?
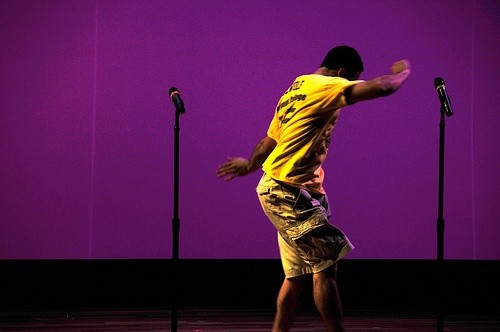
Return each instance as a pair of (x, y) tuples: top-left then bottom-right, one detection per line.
(215, 45), (411, 332)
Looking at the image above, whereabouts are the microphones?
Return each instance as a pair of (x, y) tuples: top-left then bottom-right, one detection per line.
(434, 77), (453, 116)
(169, 87), (185, 114)
